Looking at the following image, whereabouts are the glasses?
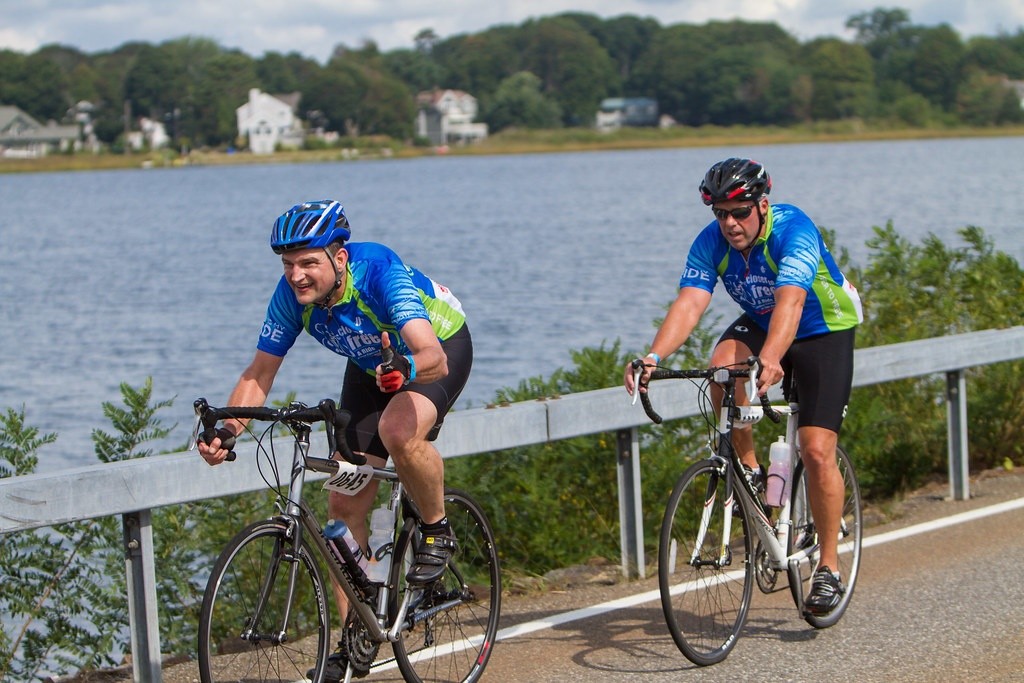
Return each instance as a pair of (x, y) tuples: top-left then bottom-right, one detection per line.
(712, 204), (756, 220)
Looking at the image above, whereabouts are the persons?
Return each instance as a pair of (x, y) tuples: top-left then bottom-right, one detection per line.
(195, 199), (474, 683)
(625, 159), (864, 612)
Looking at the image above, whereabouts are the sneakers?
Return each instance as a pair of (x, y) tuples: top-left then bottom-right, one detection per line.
(732, 464), (767, 517)
(806, 566), (845, 615)
(306, 641), (369, 680)
(405, 526), (459, 585)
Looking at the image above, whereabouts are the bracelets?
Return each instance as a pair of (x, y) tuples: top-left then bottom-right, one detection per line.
(646, 353), (661, 363)
(405, 355), (416, 385)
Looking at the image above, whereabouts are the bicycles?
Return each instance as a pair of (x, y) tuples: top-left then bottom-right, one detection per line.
(628, 358), (864, 668)
(185, 396), (501, 682)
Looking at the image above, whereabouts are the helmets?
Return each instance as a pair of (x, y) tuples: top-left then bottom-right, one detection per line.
(699, 158), (772, 206)
(270, 199), (350, 255)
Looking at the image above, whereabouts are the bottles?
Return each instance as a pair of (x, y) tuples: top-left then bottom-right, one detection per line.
(364, 503), (395, 582)
(765, 435), (791, 507)
(323, 519), (368, 571)
